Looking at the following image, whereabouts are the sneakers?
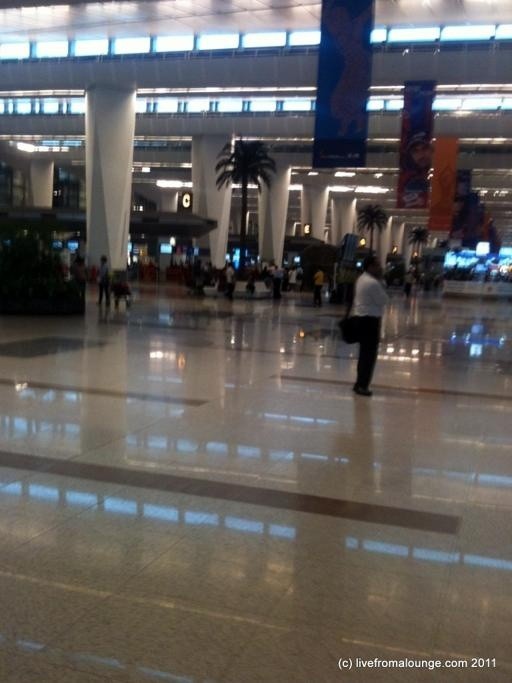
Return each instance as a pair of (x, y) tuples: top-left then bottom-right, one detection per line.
(352, 383), (373, 396)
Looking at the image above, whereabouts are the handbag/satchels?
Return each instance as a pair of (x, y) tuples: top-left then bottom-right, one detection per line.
(337, 315), (360, 344)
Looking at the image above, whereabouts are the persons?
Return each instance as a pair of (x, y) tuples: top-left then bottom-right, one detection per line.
(32, 248), (129, 315)
(128, 241), (512, 306)
(341, 255), (389, 395)
